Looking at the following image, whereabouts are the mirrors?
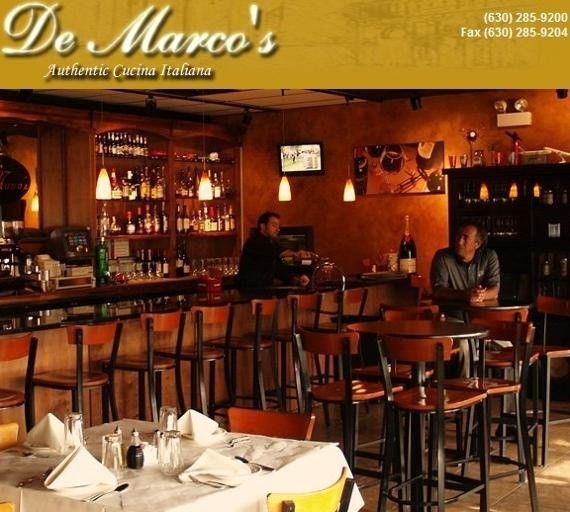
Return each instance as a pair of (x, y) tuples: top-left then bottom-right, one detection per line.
(0, 124), (69, 243)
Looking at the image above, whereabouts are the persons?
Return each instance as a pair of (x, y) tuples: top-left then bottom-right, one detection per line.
(428, 216), (502, 379)
(236, 210), (310, 291)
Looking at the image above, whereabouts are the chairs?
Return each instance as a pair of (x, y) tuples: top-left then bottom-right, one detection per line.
(1, 274), (570, 512)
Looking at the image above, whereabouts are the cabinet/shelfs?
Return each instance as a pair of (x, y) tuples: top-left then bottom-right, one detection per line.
(94, 119), (174, 271)
(174, 123), (248, 271)
(443, 160), (570, 321)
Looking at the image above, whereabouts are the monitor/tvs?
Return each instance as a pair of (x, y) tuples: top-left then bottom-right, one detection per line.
(278, 142), (324, 177)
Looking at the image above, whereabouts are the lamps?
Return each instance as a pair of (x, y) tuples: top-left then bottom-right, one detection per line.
(196, 171), (214, 204)
(276, 174), (294, 204)
(30, 192), (40, 212)
(96, 167), (114, 199)
(340, 174), (357, 203)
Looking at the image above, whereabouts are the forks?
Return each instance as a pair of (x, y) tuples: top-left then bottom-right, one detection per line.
(224, 436), (248, 445)
(225, 440), (251, 448)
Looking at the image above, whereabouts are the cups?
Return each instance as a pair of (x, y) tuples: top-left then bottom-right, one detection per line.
(362, 253), (399, 274)
(456, 180), (524, 243)
(353, 142), (443, 194)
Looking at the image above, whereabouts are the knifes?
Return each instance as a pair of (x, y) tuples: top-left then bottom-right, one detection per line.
(233, 456), (275, 472)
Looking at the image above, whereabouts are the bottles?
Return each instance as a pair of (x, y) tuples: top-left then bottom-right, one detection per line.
(438, 313), (448, 327)
(476, 283), (486, 294)
(62, 403), (186, 478)
(448, 149), (506, 170)
(396, 214), (418, 275)
(534, 188), (569, 299)
(0, 129), (248, 328)
(311, 256), (346, 292)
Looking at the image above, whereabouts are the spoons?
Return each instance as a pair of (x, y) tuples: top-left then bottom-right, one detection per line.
(5, 448), (34, 458)
(82, 482), (130, 503)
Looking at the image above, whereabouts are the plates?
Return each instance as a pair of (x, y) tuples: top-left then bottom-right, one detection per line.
(245, 463), (263, 478)
(181, 426), (228, 441)
